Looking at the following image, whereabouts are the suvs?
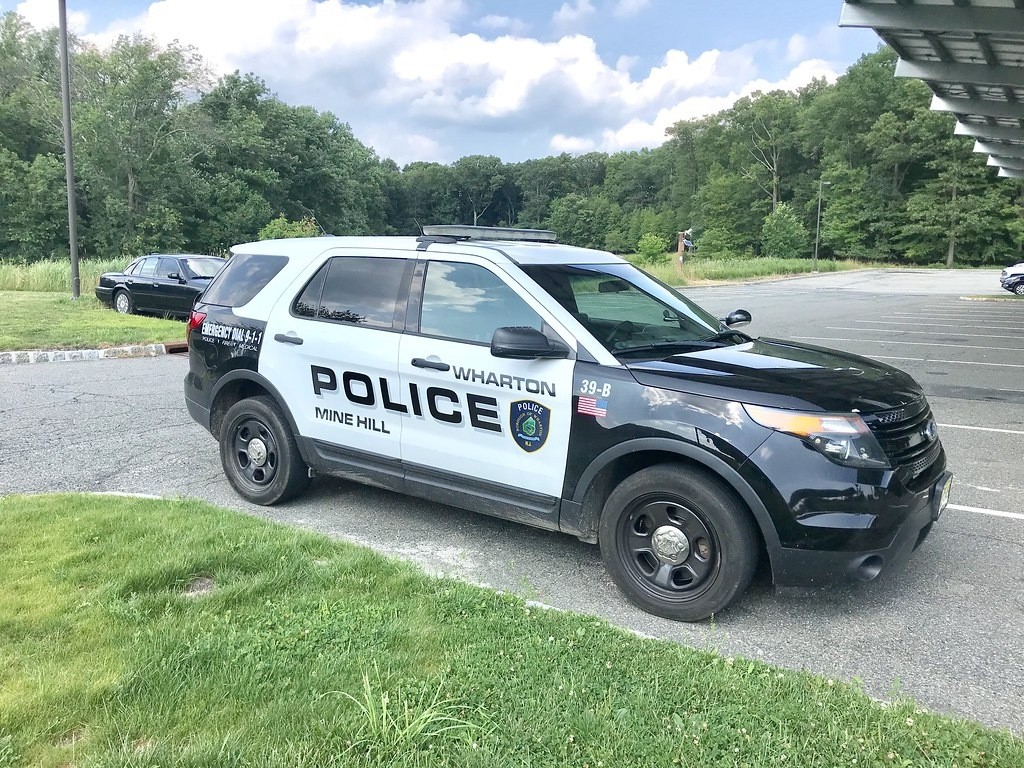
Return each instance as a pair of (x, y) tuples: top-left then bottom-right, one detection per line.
(186, 221), (956, 625)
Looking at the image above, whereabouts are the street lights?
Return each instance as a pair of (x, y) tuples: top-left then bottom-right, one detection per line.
(812, 180), (831, 273)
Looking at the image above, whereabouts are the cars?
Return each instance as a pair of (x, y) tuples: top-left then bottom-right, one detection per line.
(1001, 274), (1024, 295)
(1000, 262), (1024, 283)
(97, 253), (231, 319)
